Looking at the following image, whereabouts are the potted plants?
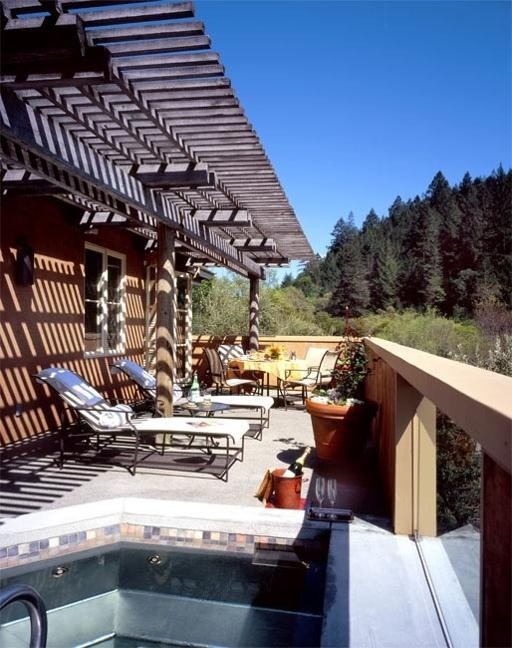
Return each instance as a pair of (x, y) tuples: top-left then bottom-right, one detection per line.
(304, 322), (381, 462)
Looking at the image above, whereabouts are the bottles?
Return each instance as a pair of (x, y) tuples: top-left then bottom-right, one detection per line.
(191, 373), (200, 402)
(282, 446), (311, 479)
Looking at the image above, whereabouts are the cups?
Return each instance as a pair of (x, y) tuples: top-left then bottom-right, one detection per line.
(187, 391), (212, 405)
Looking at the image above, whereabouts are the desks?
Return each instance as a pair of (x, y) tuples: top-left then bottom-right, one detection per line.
(179, 401), (230, 450)
(227, 354), (304, 405)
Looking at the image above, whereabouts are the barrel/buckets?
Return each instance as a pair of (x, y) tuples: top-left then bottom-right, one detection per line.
(271, 468), (304, 507)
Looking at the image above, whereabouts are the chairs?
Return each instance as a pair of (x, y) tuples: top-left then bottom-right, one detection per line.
(33, 367), (250, 481)
(204, 346), (260, 396)
(281, 346), (340, 413)
(110, 358), (275, 441)
